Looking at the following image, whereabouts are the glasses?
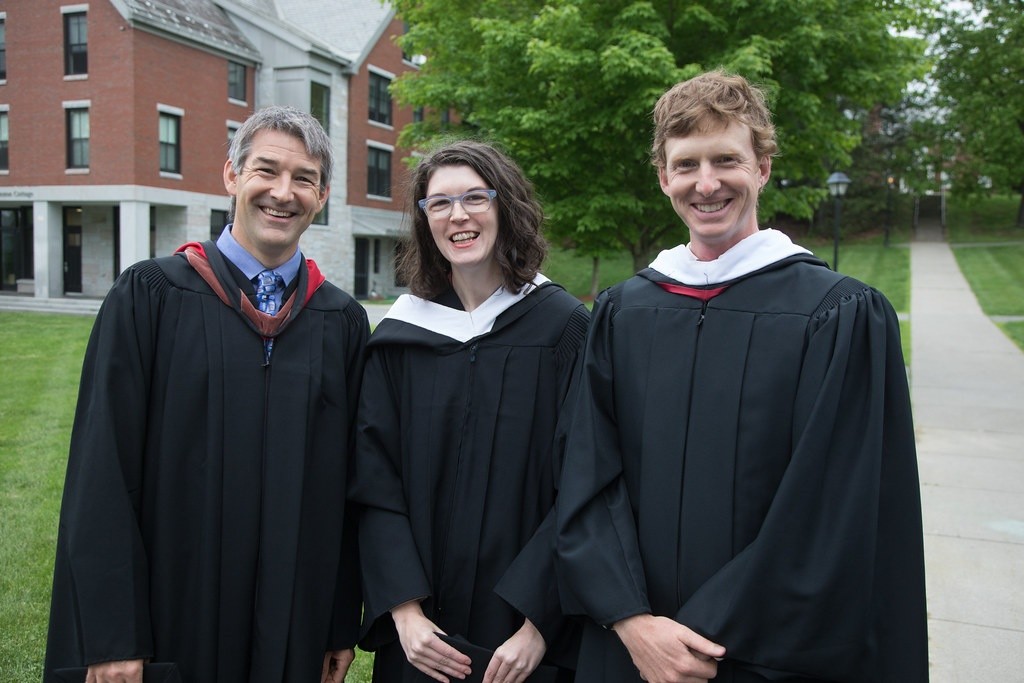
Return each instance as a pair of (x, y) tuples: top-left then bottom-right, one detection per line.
(417, 190), (497, 220)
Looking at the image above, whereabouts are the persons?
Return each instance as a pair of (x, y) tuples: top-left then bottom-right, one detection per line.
(41, 102), (374, 682)
(352, 137), (592, 683)
(547, 63), (933, 683)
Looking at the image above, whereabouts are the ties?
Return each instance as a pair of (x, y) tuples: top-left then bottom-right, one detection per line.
(255, 270), (283, 359)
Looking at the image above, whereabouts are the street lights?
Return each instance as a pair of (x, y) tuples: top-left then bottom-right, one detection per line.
(824, 171), (853, 272)
